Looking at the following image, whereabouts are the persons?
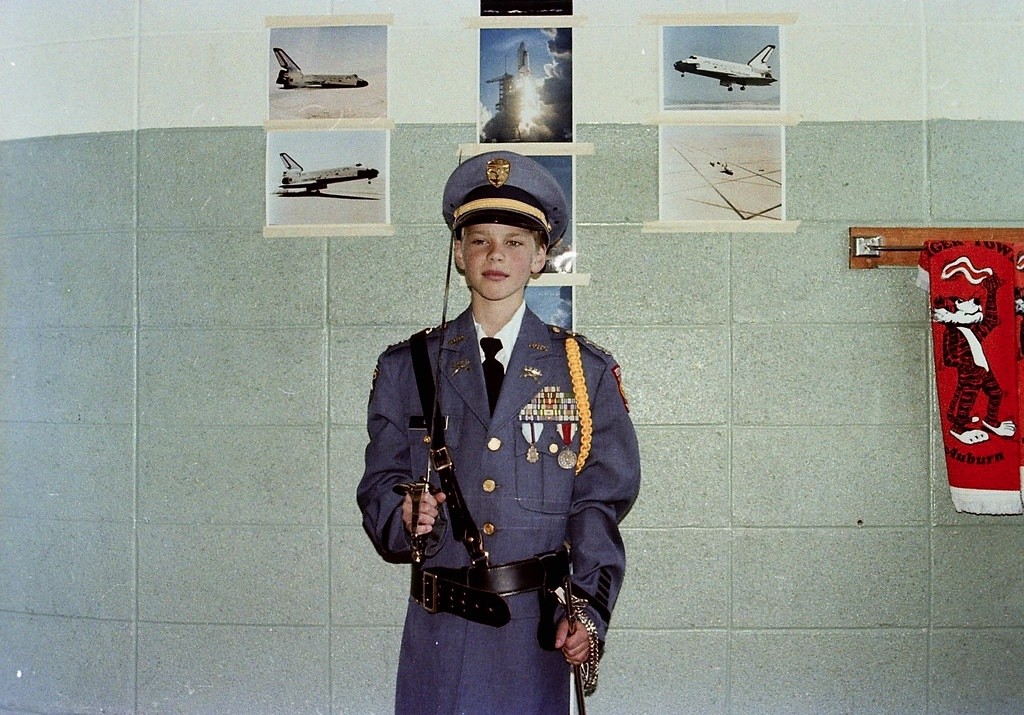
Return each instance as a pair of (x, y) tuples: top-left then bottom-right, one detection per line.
(357, 149), (641, 715)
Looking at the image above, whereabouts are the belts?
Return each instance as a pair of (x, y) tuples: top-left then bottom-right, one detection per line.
(411, 548), (569, 628)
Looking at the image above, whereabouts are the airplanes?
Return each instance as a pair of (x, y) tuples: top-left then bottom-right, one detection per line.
(279, 151), (377, 195)
(673, 43), (779, 92)
(273, 46), (370, 89)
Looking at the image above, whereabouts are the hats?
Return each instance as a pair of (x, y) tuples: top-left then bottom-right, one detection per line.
(442, 151), (569, 250)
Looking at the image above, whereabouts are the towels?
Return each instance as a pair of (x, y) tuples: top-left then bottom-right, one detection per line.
(916, 239), (1023, 515)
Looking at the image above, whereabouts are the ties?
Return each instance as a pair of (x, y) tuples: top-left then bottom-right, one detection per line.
(480, 337), (505, 419)
